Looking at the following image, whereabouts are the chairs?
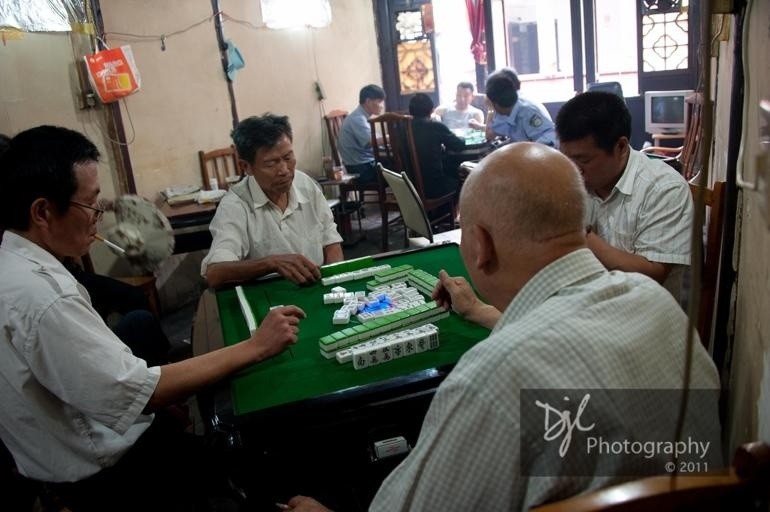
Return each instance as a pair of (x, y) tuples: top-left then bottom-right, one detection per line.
(323, 109), (464, 252)
(639, 94), (715, 179)
(513, 439), (770, 511)
(666, 176), (726, 351)
(198, 142), (243, 194)
(76, 249), (161, 319)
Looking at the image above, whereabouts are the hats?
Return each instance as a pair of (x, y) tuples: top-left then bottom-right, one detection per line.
(101, 194), (178, 278)
(224, 37), (247, 85)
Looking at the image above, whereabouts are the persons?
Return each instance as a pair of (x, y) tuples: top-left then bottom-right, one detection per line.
(201, 110), (345, 288)
(337, 69), (560, 231)
(288, 141), (721, 512)
(556, 92), (694, 305)
(94, 233), (125, 254)
(0, 124), (306, 511)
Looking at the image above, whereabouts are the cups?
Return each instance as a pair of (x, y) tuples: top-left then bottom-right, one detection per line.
(225, 176), (241, 189)
(333, 165), (345, 181)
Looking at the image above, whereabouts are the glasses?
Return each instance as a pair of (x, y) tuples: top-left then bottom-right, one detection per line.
(46, 192), (106, 224)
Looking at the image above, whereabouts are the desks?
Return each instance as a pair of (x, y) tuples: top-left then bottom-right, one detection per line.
(151, 191), (223, 254)
(189, 227), (507, 512)
(380, 126), (511, 161)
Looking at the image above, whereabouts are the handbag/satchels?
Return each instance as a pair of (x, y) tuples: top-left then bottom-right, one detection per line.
(82, 34), (144, 105)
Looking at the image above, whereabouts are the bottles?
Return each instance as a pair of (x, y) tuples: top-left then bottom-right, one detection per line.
(323, 156), (336, 179)
(208, 178), (218, 190)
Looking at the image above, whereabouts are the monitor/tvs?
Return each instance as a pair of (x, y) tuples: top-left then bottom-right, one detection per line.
(644, 89), (694, 135)
